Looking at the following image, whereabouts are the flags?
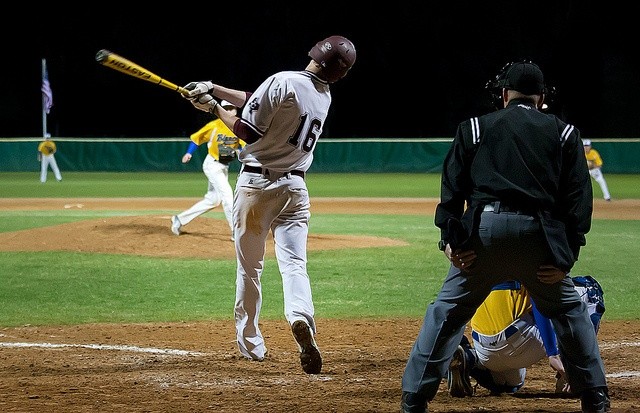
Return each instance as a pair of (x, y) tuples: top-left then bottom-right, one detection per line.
(41, 61), (55, 114)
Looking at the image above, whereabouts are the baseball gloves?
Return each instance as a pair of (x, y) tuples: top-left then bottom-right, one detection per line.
(218, 145), (236, 163)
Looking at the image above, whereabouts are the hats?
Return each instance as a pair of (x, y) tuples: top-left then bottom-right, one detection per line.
(583, 139), (590, 147)
(220, 99), (236, 108)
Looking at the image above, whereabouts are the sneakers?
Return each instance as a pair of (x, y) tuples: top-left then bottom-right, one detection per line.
(554, 374), (572, 395)
(447, 345), (473, 397)
(291, 319), (322, 374)
(170, 215), (181, 236)
(581, 387), (610, 412)
(401, 392), (428, 412)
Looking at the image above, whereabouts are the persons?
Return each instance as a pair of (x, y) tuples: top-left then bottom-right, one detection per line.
(449, 276), (606, 399)
(180, 35), (355, 372)
(400, 60), (609, 411)
(582, 137), (612, 200)
(37, 133), (63, 183)
(169, 98), (235, 241)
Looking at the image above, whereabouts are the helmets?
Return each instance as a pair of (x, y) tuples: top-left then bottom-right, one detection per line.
(485, 60), (556, 107)
(308, 36), (356, 81)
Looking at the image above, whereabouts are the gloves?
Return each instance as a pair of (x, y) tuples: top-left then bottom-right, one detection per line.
(181, 81), (214, 101)
(188, 94), (219, 114)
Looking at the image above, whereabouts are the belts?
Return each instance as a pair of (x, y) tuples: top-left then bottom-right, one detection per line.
(482, 203), (547, 212)
(242, 164), (304, 179)
(471, 320), (526, 345)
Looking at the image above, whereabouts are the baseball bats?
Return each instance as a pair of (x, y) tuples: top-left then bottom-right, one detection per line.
(95, 49), (196, 98)
